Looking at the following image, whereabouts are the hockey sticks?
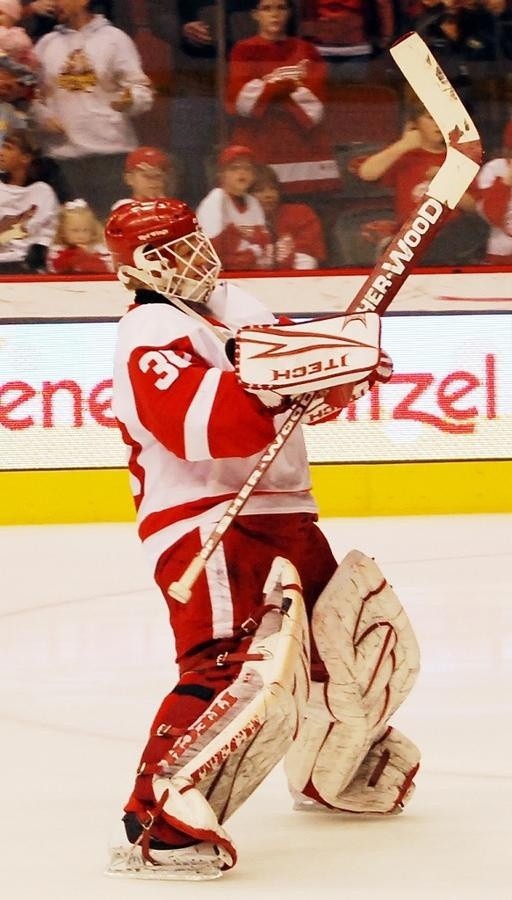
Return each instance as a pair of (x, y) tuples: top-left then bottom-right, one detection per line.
(168, 31), (482, 603)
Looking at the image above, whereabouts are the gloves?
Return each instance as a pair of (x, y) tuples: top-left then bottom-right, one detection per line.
(234, 313), (393, 427)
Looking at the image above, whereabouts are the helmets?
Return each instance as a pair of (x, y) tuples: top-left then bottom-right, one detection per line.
(106, 198), (203, 291)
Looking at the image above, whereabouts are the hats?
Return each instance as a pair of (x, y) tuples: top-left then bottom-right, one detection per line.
(215, 145), (258, 166)
(124, 146), (173, 172)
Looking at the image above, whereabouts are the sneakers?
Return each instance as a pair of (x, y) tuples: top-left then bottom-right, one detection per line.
(123, 804), (204, 850)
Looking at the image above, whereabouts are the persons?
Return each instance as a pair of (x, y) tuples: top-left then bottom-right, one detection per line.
(104, 198), (421, 871)
